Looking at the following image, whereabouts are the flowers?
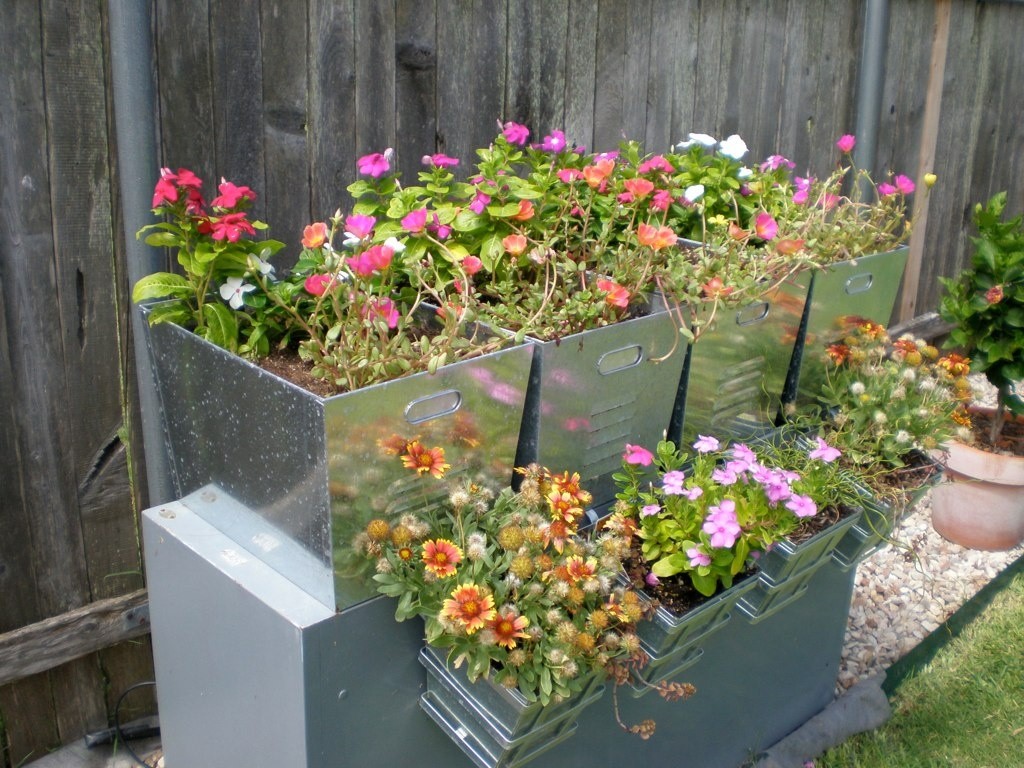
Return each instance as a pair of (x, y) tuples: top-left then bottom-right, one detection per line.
(132, 121), (1024, 742)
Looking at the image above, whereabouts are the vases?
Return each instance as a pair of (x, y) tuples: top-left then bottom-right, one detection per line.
(930, 407), (1024, 550)
(417, 615), (617, 768)
(829, 442), (944, 574)
(485, 270), (698, 527)
(610, 246), (814, 456)
(575, 534), (763, 700)
(785, 242), (907, 420)
(141, 294), (535, 612)
(702, 452), (866, 627)
